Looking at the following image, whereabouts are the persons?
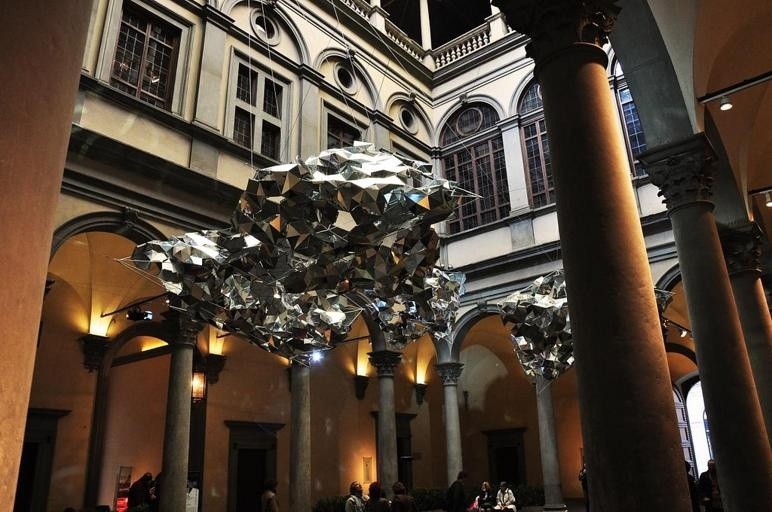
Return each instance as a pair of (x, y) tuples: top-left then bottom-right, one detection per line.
(64, 505), (118, 512)
(344, 481), (417, 512)
(685, 460), (724, 512)
(126, 473), (199, 512)
(259, 479), (281, 511)
(440, 471), (518, 512)
(577, 463), (590, 512)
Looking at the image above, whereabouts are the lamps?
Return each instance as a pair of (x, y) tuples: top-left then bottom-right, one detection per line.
(353, 374), (370, 399)
(415, 382), (428, 405)
(75, 331), (112, 375)
(191, 353), (227, 403)
